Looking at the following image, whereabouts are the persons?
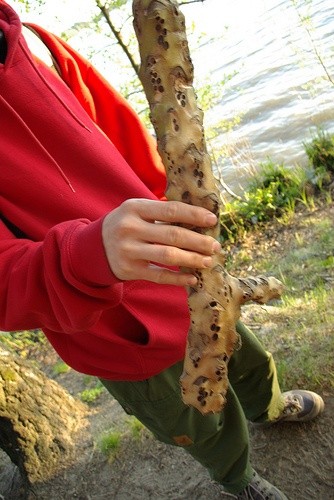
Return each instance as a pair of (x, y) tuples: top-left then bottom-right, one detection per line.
(0, 0), (326, 500)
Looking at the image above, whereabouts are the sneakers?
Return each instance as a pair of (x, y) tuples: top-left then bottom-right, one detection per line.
(219, 468), (288, 500)
(248, 389), (325, 426)
